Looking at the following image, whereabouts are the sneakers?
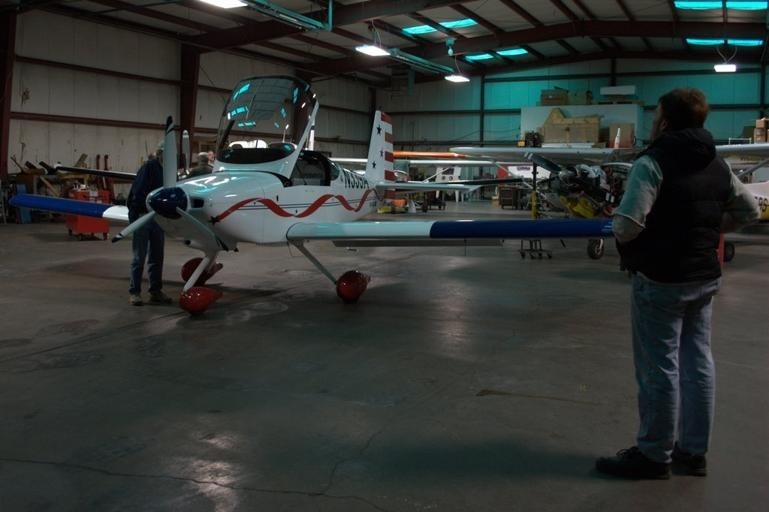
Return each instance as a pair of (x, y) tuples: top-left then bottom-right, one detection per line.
(148, 293), (173, 305)
(596, 448), (672, 479)
(669, 443), (708, 475)
(130, 296), (143, 306)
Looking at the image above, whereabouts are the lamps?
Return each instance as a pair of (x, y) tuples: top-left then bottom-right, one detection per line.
(354, 24), (390, 57)
(238, 0), (334, 32)
(387, 48), (454, 75)
(444, 50), (471, 83)
(445, 37), (457, 56)
(713, 33), (740, 73)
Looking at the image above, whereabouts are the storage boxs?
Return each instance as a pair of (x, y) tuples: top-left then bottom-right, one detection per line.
(609, 122), (634, 148)
(743, 117), (769, 143)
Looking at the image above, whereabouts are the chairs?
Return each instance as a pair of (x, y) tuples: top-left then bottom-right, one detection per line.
(268, 142), (296, 154)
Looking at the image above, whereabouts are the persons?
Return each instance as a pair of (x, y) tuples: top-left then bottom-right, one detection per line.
(593, 87), (762, 483)
(186, 150), (213, 178)
(126, 140), (173, 306)
(206, 152), (216, 161)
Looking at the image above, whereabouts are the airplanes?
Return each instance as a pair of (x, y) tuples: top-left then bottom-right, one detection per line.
(48, 127), (194, 185)
(392, 145), (525, 211)
(447, 139), (769, 270)
(6, 71), (619, 318)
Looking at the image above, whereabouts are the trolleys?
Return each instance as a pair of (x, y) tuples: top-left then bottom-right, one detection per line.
(59, 183), (115, 243)
(497, 184), (517, 211)
